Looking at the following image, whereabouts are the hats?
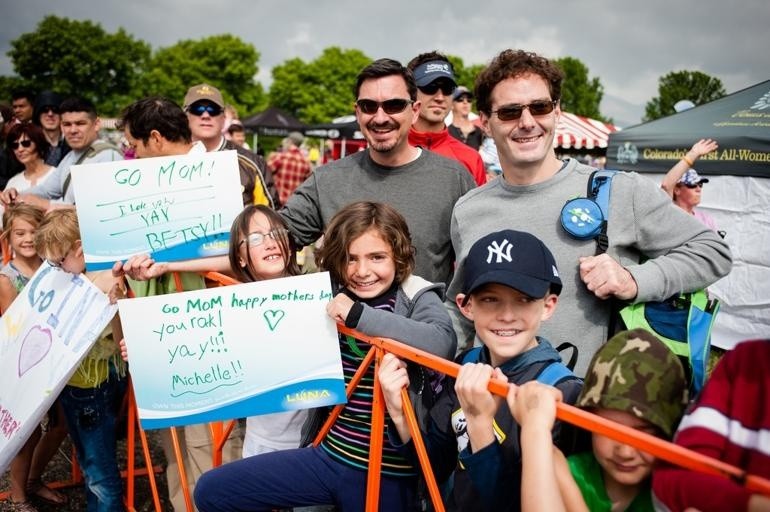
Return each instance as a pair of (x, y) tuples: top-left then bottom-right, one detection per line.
(461, 229), (563, 309)
(452, 85), (473, 102)
(676, 168), (709, 186)
(31, 92), (62, 127)
(572, 325), (686, 443)
(183, 82), (225, 113)
(281, 131), (305, 147)
(412, 60), (459, 87)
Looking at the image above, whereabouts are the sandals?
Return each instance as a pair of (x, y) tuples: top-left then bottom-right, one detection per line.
(0, 494), (46, 512)
(24, 477), (70, 508)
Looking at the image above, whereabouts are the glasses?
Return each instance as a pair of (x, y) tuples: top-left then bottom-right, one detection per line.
(455, 96), (473, 103)
(417, 85), (456, 96)
(9, 140), (32, 150)
(186, 104), (224, 117)
(237, 227), (291, 251)
(40, 106), (61, 114)
(678, 183), (704, 189)
(487, 99), (557, 121)
(356, 99), (415, 116)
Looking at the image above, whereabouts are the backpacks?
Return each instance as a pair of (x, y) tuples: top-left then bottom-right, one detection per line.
(559, 168), (722, 400)
